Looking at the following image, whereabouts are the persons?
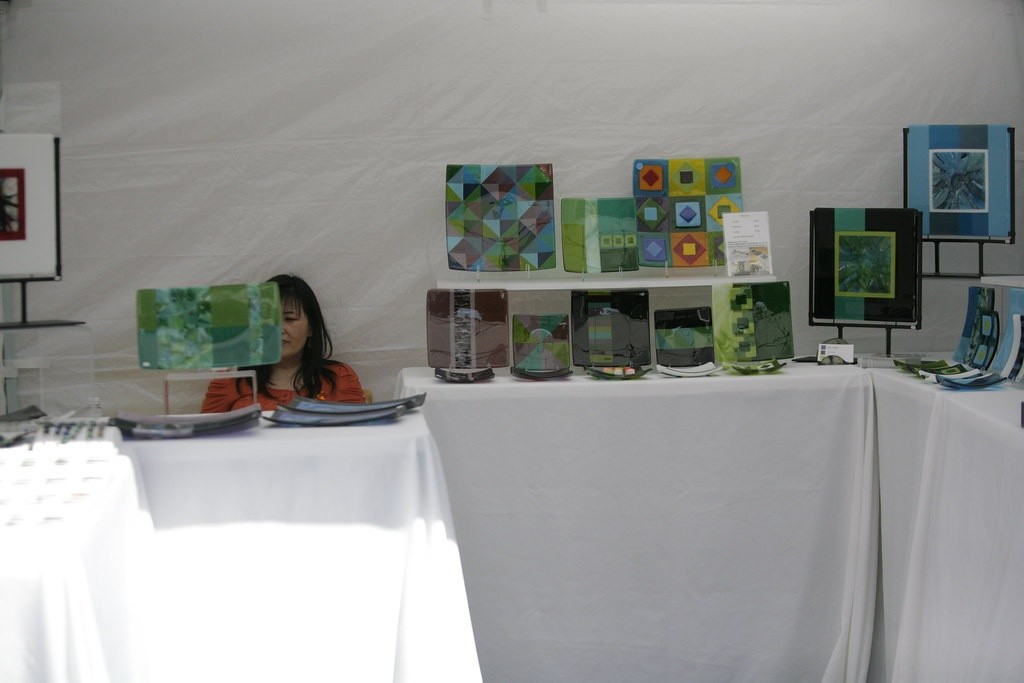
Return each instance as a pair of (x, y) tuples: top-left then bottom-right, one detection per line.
(201, 274), (366, 412)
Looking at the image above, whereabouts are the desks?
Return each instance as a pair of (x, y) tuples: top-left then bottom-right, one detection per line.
(0, 352), (1024, 683)
